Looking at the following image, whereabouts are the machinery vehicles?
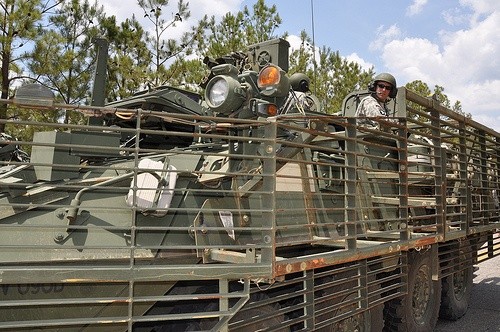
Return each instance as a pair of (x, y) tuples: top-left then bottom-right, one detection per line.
(1, 33), (500, 332)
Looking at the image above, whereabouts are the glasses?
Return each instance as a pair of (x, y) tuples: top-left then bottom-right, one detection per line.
(376, 84), (392, 90)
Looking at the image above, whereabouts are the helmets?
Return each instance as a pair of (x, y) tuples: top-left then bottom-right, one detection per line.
(289, 73), (310, 92)
(373, 73), (396, 87)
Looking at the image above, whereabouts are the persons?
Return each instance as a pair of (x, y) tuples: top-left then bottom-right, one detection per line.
(286, 73), (321, 113)
(353, 73), (398, 135)
